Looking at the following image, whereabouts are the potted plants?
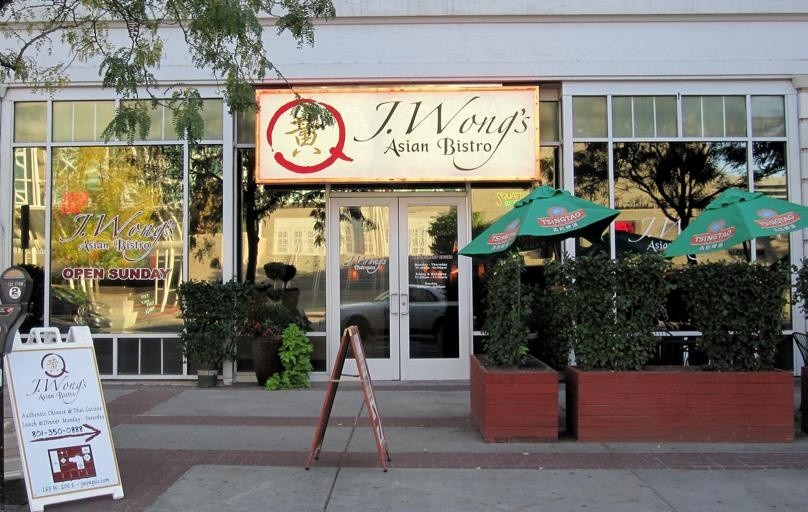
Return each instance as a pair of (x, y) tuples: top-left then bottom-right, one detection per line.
(253, 263), (313, 387)
(470, 252), (559, 444)
(547, 251), (794, 442)
(176, 279), (261, 387)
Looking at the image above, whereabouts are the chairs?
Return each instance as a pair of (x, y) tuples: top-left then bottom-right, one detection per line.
(792, 333), (808, 413)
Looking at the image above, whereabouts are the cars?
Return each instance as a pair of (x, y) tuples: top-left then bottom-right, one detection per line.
(319, 284), (449, 347)
(49, 284), (113, 335)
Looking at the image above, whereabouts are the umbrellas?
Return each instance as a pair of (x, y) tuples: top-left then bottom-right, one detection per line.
(655, 183), (807, 288)
(451, 183), (622, 371)
